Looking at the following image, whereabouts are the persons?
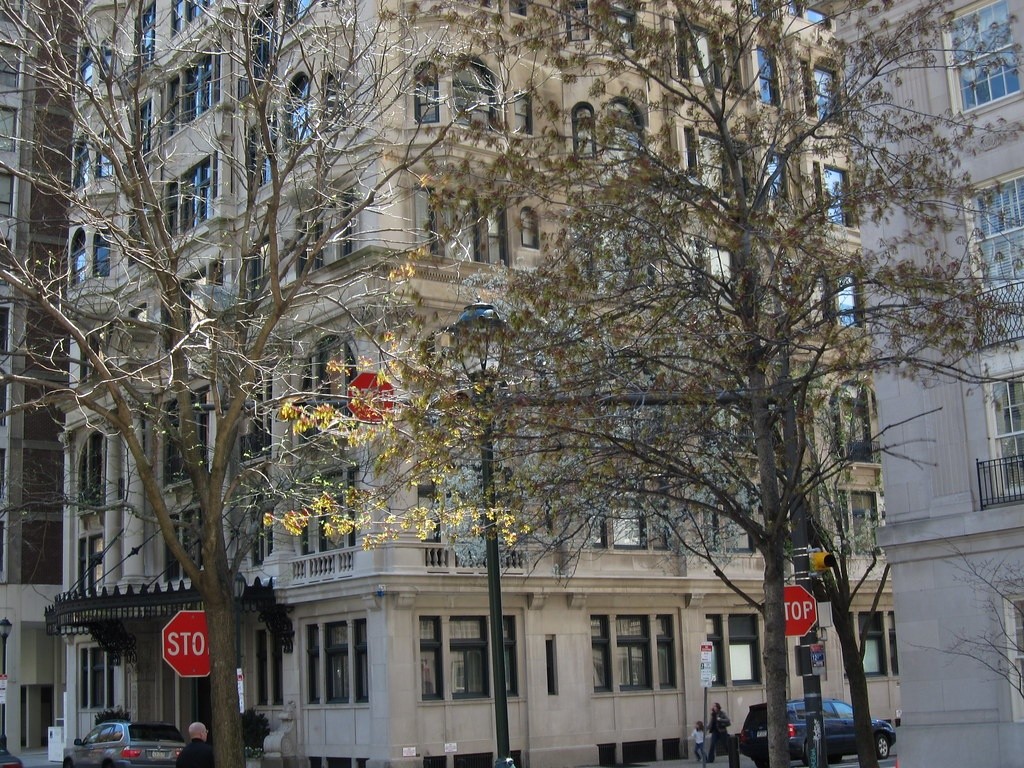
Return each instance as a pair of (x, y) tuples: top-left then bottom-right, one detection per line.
(687, 721), (708, 762)
(176, 722), (215, 768)
(707, 703), (731, 762)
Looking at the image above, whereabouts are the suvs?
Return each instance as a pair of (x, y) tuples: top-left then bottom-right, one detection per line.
(63, 720), (186, 768)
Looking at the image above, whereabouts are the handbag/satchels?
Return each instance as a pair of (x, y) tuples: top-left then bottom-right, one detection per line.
(719, 713), (731, 727)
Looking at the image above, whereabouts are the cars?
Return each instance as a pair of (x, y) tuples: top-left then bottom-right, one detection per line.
(738, 699), (896, 768)
(0, 734), (23, 768)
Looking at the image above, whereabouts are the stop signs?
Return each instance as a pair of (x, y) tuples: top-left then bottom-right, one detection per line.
(346, 373), (395, 424)
(784, 585), (817, 637)
(162, 611), (212, 677)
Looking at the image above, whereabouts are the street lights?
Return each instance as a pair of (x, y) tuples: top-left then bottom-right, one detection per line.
(447, 296), (520, 768)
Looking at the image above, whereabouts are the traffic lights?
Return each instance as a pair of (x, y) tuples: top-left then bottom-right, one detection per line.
(811, 553), (837, 571)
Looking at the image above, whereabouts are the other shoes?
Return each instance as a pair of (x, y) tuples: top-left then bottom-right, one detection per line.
(707, 759), (714, 762)
(697, 757), (701, 761)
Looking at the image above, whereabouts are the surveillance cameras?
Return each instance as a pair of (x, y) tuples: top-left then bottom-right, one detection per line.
(813, 553), (836, 570)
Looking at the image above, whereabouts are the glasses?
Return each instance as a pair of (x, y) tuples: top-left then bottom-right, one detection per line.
(204, 730), (209, 733)
